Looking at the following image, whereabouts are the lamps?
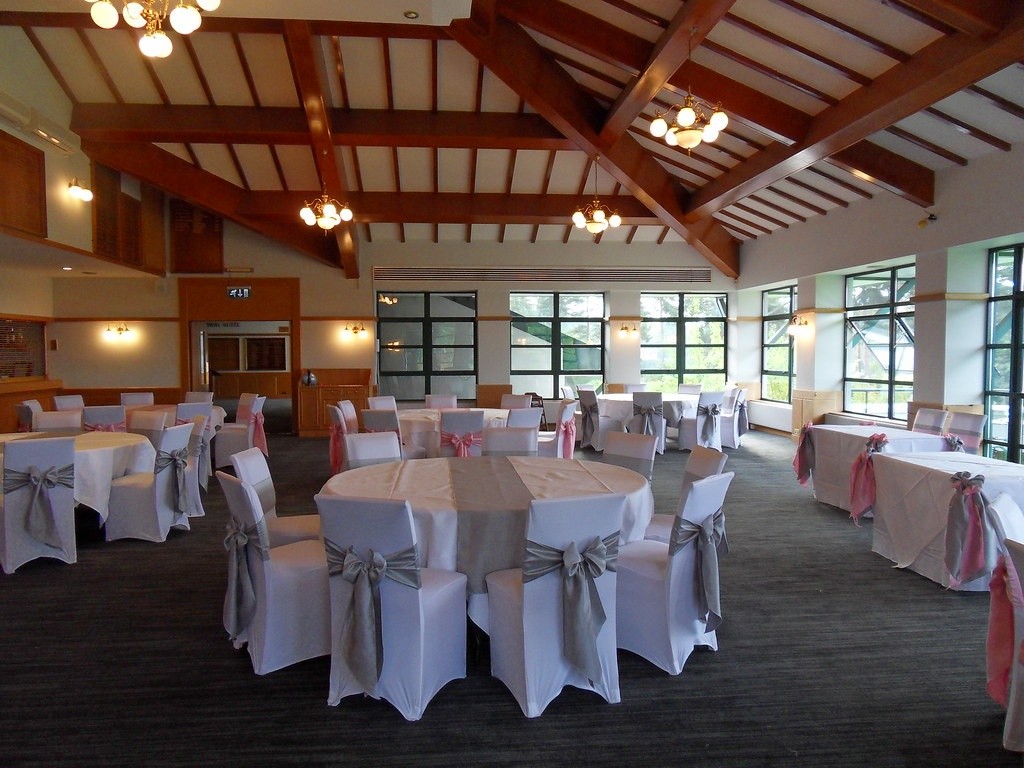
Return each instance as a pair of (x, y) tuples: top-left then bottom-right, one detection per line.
(344, 322), (365, 334)
(105, 321), (130, 336)
(571, 163), (621, 242)
(300, 148), (353, 230)
(84, 0), (221, 58)
(67, 176), (93, 201)
(650, 25), (729, 157)
(787, 315), (809, 335)
(619, 322), (638, 338)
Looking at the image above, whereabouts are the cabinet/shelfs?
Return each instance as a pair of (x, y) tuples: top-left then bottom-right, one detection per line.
(296, 383), (369, 438)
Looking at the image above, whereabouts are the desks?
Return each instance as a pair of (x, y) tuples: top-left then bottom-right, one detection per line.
(125, 404), (178, 433)
(807, 425), (952, 518)
(869, 450), (1024, 589)
(396, 408), (509, 456)
(597, 394), (699, 435)
(0, 431), (156, 529)
(319, 457), (652, 598)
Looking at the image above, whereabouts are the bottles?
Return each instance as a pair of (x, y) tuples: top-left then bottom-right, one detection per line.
(307, 370), (312, 386)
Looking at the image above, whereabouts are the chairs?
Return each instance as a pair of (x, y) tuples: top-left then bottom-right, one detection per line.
(0, 379), (1024, 752)
